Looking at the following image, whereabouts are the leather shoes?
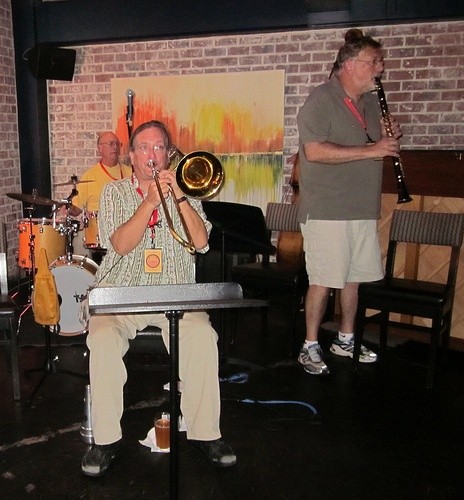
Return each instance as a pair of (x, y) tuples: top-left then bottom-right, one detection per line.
(82, 441), (121, 476)
(194, 437), (236, 468)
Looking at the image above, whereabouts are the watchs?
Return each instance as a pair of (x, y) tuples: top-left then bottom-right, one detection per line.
(178, 195), (188, 203)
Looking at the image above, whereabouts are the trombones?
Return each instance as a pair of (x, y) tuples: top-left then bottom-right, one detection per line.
(148, 144), (224, 254)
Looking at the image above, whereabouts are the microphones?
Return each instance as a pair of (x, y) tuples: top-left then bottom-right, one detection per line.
(126, 89), (135, 118)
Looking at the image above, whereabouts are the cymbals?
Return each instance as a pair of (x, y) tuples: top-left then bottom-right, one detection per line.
(54, 180), (95, 186)
(6, 193), (56, 205)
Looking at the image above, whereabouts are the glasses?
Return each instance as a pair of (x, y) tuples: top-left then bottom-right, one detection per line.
(101, 141), (121, 148)
(354, 59), (385, 66)
(133, 145), (167, 155)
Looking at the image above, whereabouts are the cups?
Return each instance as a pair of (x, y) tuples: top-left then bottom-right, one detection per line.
(154, 412), (170, 449)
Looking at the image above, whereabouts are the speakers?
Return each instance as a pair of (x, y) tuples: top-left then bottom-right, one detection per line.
(27, 45), (76, 82)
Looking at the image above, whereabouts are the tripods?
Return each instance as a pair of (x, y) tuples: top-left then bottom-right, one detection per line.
(23, 325), (89, 409)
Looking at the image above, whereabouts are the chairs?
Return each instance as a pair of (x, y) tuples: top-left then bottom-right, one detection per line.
(229, 202), (335, 349)
(353, 210), (464, 390)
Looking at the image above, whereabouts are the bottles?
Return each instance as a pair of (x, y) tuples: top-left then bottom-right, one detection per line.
(220, 372), (249, 384)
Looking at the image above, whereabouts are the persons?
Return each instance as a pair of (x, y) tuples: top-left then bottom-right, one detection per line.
(62, 132), (134, 265)
(80, 121), (237, 475)
(296, 36), (402, 373)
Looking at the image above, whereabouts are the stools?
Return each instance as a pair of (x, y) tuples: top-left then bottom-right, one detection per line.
(0, 294), (20, 400)
(122, 325), (178, 376)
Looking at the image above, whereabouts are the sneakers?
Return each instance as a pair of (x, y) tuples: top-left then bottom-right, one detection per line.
(298, 344), (328, 375)
(331, 336), (378, 363)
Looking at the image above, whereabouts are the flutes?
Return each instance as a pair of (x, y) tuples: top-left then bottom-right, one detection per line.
(373, 75), (414, 204)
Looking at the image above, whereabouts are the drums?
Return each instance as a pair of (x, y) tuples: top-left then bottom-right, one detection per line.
(83, 211), (108, 251)
(15, 218), (73, 271)
(30, 254), (99, 336)
